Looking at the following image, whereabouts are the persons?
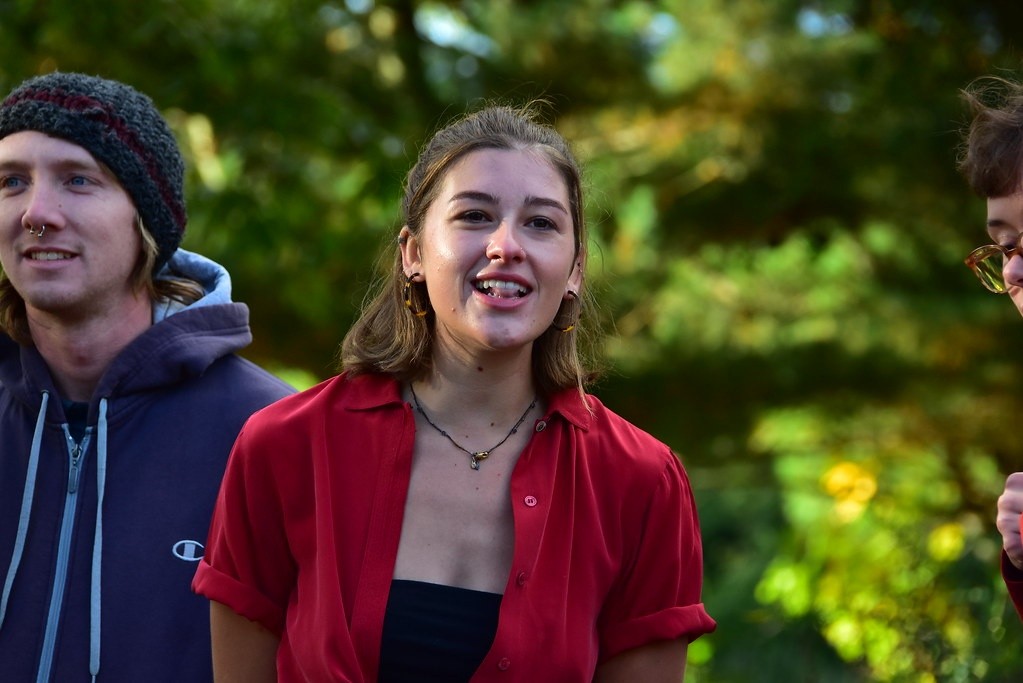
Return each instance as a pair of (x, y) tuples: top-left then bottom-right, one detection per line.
(190, 101), (716, 682)
(957, 77), (1023, 624)
(0, 72), (306, 683)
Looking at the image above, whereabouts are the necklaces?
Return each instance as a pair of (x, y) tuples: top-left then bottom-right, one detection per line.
(409, 382), (541, 469)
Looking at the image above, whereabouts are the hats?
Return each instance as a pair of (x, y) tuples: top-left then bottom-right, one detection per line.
(0, 73), (186, 279)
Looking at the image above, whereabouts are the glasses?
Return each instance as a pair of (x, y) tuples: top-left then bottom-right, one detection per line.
(963, 232), (1023, 293)
